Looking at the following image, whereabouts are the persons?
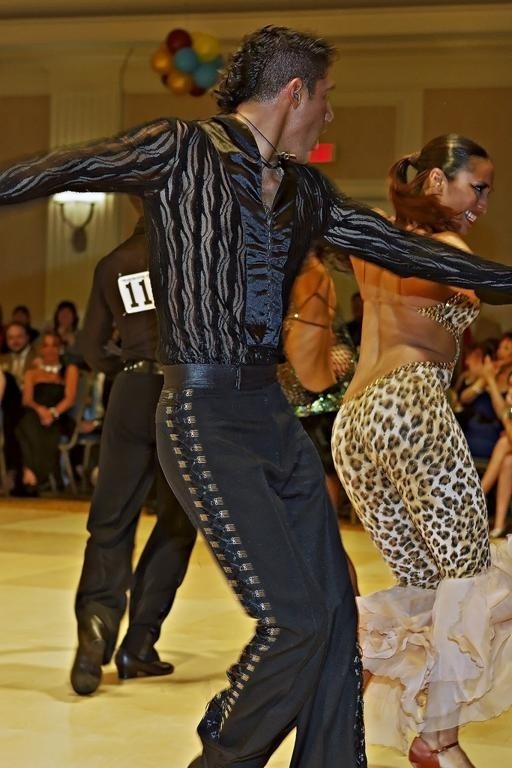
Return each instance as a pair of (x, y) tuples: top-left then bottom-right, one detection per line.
(327, 130), (494, 767)
(0, 22), (512, 767)
(61, 195), (199, 694)
(283, 243), (355, 516)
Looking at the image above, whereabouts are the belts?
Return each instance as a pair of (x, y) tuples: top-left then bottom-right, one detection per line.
(121, 359), (168, 376)
(160, 362), (278, 390)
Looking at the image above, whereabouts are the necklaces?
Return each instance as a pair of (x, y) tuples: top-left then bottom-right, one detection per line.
(236, 109), (298, 170)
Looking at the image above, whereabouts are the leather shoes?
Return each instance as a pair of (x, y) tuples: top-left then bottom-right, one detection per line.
(69, 613), (107, 697)
(407, 735), (460, 768)
(113, 648), (178, 681)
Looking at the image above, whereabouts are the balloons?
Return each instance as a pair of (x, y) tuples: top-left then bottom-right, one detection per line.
(153, 25), (225, 96)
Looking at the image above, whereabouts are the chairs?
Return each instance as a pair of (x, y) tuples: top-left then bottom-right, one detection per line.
(42, 367), (105, 496)
